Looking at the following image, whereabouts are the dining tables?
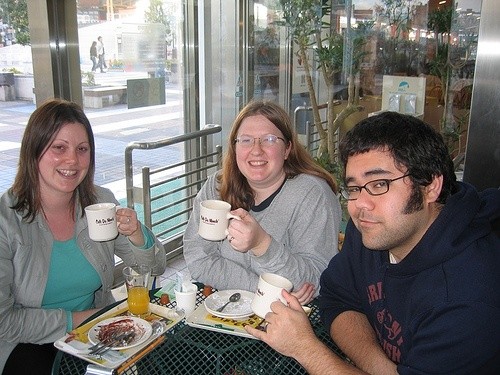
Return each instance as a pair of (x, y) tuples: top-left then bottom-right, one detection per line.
(52, 282), (356, 375)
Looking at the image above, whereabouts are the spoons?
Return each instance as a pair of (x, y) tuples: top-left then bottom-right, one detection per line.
(217, 293), (241, 312)
(97, 329), (135, 355)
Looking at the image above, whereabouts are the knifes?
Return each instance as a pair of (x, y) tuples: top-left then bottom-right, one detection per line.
(89, 332), (124, 355)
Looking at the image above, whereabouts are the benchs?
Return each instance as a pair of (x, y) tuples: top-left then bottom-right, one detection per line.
(83, 86), (127, 108)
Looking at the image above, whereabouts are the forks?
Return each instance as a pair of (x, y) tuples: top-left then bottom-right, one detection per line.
(87, 327), (122, 351)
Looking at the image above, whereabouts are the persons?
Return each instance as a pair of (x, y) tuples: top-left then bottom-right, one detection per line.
(256, 42), (278, 103)
(183, 102), (339, 306)
(90, 36), (107, 73)
(0, 99), (166, 375)
(245, 111), (500, 375)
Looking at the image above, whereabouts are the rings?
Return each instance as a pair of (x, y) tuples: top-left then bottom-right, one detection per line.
(229, 237), (235, 243)
(127, 217), (130, 223)
(242, 212), (251, 219)
(263, 322), (269, 331)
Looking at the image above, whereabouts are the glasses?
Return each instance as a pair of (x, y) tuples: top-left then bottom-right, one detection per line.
(231, 132), (286, 147)
(340, 172), (410, 201)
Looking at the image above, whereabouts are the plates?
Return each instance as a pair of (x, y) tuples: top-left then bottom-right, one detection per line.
(88, 316), (153, 348)
(204, 290), (256, 319)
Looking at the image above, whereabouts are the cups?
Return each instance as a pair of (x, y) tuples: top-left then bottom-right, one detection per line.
(123, 264), (151, 316)
(174, 284), (197, 318)
(85, 203), (119, 242)
(251, 273), (293, 320)
(199, 200), (242, 242)
